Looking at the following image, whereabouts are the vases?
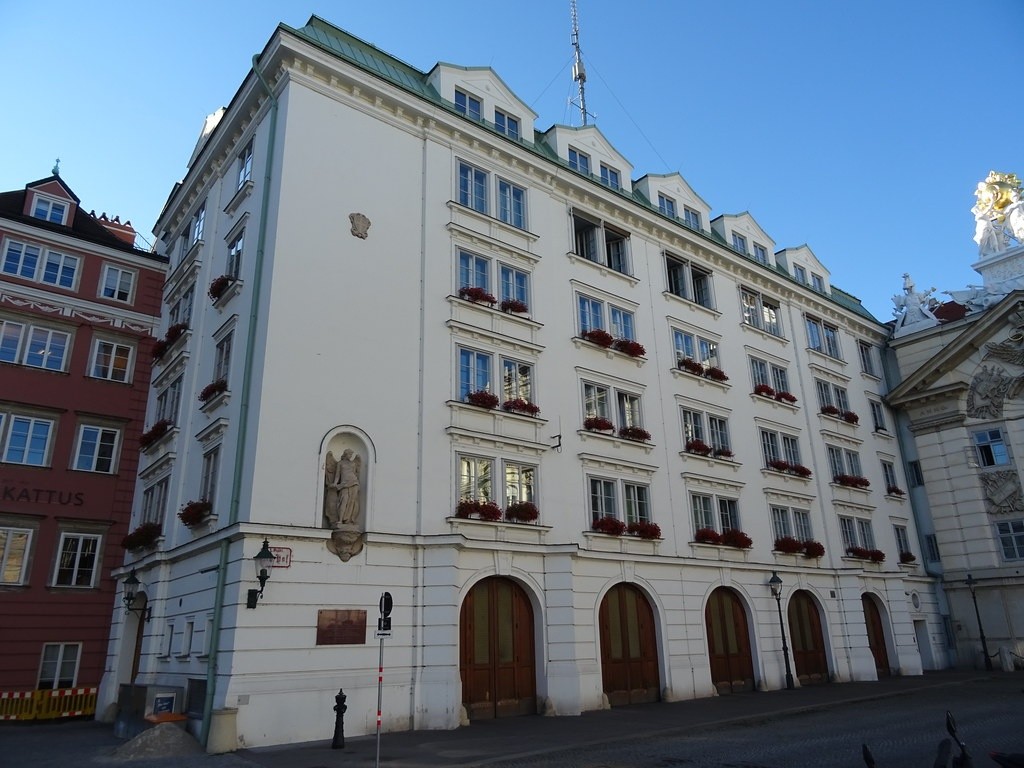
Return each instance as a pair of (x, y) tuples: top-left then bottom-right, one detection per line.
(470, 401), (496, 410)
(472, 300), (530, 322)
(855, 484), (869, 491)
(467, 511), (530, 526)
(759, 392), (796, 407)
(588, 429), (615, 436)
(770, 467), (803, 480)
(623, 436), (648, 445)
(507, 408), (534, 418)
(205, 391), (219, 402)
(681, 365), (723, 384)
(827, 412), (839, 419)
(691, 450), (734, 465)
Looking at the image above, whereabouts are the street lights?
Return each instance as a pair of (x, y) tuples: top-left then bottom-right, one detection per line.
(769, 570), (796, 691)
(965, 574), (993, 669)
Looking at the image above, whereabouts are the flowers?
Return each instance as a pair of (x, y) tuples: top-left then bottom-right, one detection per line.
(581, 329), (647, 356)
(584, 418), (652, 440)
(694, 528), (752, 550)
(846, 548), (887, 564)
(454, 500), (540, 523)
(149, 323), (190, 357)
(591, 518), (661, 540)
(206, 277), (228, 302)
(680, 358), (729, 380)
(686, 440), (737, 458)
(196, 380), (227, 403)
(833, 475), (871, 488)
(875, 423), (917, 565)
(139, 418), (175, 449)
(769, 461), (812, 476)
(820, 405), (859, 424)
(773, 538), (825, 559)
(120, 499), (216, 552)
(464, 391), (541, 414)
(755, 384), (798, 403)
(457, 285), (530, 311)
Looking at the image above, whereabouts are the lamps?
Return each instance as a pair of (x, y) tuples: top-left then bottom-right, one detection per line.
(121, 565), (152, 623)
(247, 536), (277, 609)
